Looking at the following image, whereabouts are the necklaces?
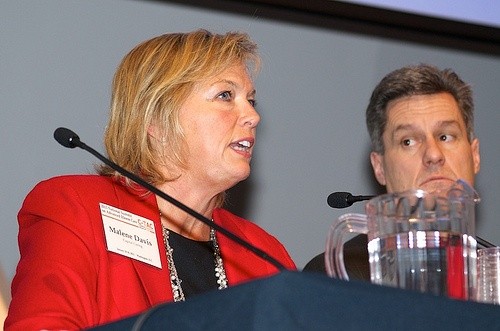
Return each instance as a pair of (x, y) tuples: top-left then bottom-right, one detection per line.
(160, 211), (228, 303)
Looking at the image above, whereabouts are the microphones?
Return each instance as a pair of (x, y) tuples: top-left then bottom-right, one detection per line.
(328, 192), (496, 248)
(54, 128), (286, 274)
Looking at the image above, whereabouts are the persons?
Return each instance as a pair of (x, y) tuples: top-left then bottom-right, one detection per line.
(302, 63), (497, 284)
(2, 29), (297, 331)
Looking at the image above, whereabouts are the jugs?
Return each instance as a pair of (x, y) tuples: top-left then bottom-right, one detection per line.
(325, 179), (480, 301)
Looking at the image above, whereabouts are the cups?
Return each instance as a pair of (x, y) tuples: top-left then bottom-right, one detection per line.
(475, 246), (500, 304)
